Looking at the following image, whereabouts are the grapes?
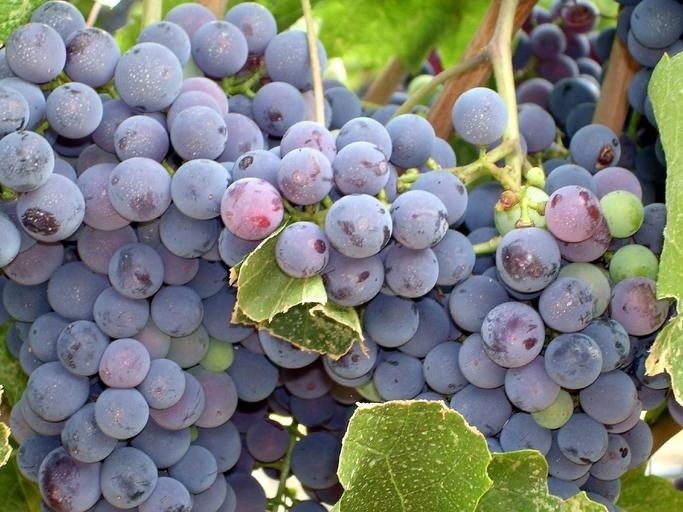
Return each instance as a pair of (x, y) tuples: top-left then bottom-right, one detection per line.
(0, 0), (683, 512)
